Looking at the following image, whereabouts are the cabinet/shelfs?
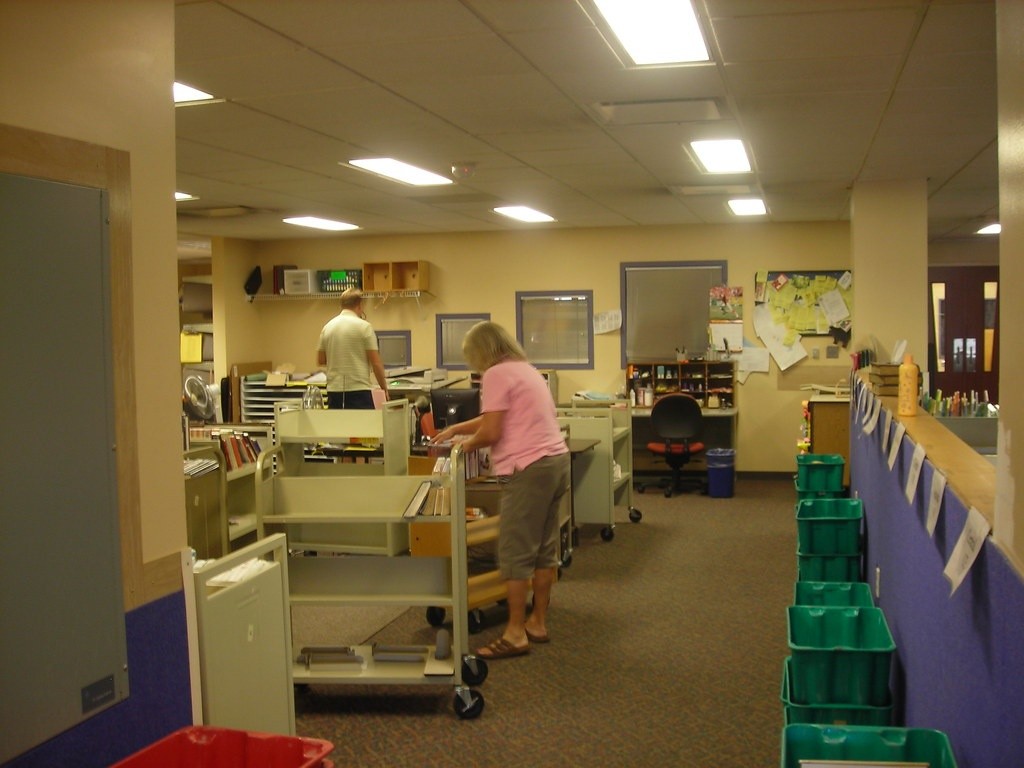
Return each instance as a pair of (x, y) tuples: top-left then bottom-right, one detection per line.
(361, 260), (429, 292)
(184, 370), (642, 738)
(808, 393), (850, 484)
(625, 361), (739, 481)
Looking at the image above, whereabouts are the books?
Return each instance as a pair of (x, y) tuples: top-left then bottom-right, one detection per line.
(432, 449), (479, 479)
(183, 416), (261, 471)
(402, 481), (450, 518)
(869, 362), (923, 396)
(465, 507), (486, 520)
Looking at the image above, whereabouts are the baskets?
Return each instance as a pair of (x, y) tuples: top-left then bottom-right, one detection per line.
(316, 269), (362, 292)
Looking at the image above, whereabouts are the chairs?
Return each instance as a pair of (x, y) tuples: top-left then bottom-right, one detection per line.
(637, 392), (707, 498)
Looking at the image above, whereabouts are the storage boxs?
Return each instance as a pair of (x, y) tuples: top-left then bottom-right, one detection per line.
(779, 455), (959, 768)
(109, 725), (332, 768)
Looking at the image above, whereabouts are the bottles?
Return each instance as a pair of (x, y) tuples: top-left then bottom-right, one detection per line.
(628, 366), (728, 409)
(314, 386), (324, 409)
(898, 355), (919, 416)
(302, 385), (314, 409)
(705, 344), (718, 360)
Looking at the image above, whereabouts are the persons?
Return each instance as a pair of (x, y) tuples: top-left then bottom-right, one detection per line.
(429, 321), (570, 659)
(315, 288), (389, 409)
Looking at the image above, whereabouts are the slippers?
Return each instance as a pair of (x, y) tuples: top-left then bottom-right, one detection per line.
(477, 636), (530, 659)
(524, 620), (549, 643)
